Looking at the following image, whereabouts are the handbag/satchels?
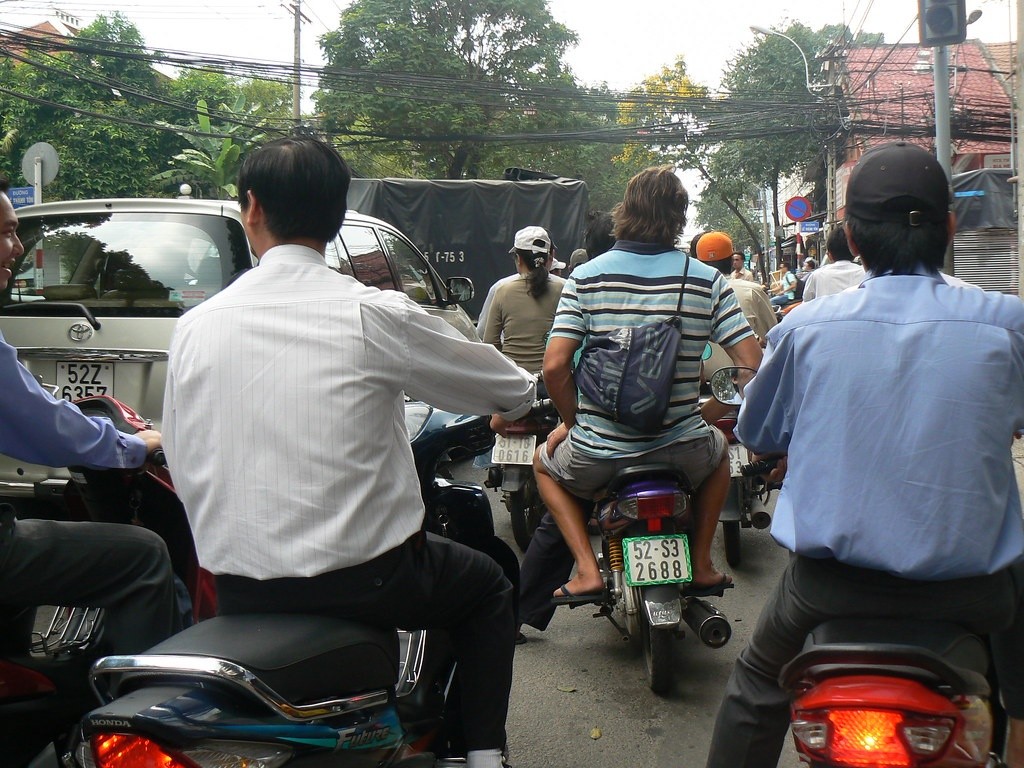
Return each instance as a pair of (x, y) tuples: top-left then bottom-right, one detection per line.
(786, 273), (805, 300)
(573, 252), (691, 434)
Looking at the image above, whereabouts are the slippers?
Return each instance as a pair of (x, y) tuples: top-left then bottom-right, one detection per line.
(696, 573), (734, 597)
(550, 582), (609, 604)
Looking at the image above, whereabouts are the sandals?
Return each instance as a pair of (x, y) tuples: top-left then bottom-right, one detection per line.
(588, 512), (602, 535)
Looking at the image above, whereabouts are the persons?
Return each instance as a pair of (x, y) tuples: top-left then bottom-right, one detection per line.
(704, 142), (1024, 768)
(470, 224), (875, 491)
(0, 172), (185, 696)
(158, 136), (551, 768)
(531, 168), (764, 605)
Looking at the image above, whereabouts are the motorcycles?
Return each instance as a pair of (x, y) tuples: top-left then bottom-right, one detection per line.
(760, 283), (800, 310)
(707, 364), (1009, 768)
(483, 373), (561, 552)
(1, 372), (219, 768)
(70, 398), (556, 768)
(694, 373), (786, 570)
(525, 328), (737, 699)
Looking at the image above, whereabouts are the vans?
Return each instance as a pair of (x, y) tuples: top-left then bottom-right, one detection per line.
(1, 196), (482, 484)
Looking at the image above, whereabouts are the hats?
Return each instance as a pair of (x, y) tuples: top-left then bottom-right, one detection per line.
(509, 226), (551, 254)
(549, 257), (567, 271)
(570, 248), (589, 269)
(543, 229), (558, 249)
(696, 232), (735, 261)
(846, 141), (954, 228)
